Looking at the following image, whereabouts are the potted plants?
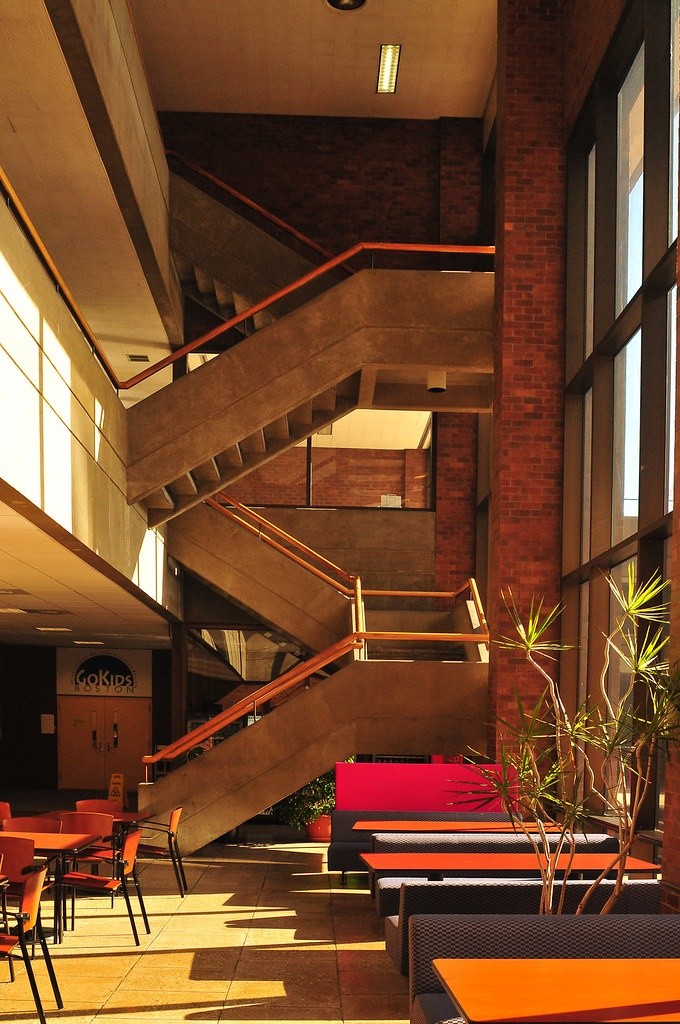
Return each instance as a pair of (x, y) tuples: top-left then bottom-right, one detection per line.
(273, 772), (336, 842)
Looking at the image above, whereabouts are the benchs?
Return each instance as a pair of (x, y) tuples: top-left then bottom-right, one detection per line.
(326, 809), (680, 1024)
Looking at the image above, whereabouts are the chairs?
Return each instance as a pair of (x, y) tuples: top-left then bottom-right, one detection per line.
(0, 799), (189, 1024)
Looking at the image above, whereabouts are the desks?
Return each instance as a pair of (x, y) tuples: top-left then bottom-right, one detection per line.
(358, 853), (661, 881)
(0, 831), (102, 944)
(352, 821), (571, 833)
(432, 958), (680, 1024)
(36, 810), (157, 896)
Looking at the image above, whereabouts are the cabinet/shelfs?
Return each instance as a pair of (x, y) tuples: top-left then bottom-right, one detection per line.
(56, 698), (154, 790)
